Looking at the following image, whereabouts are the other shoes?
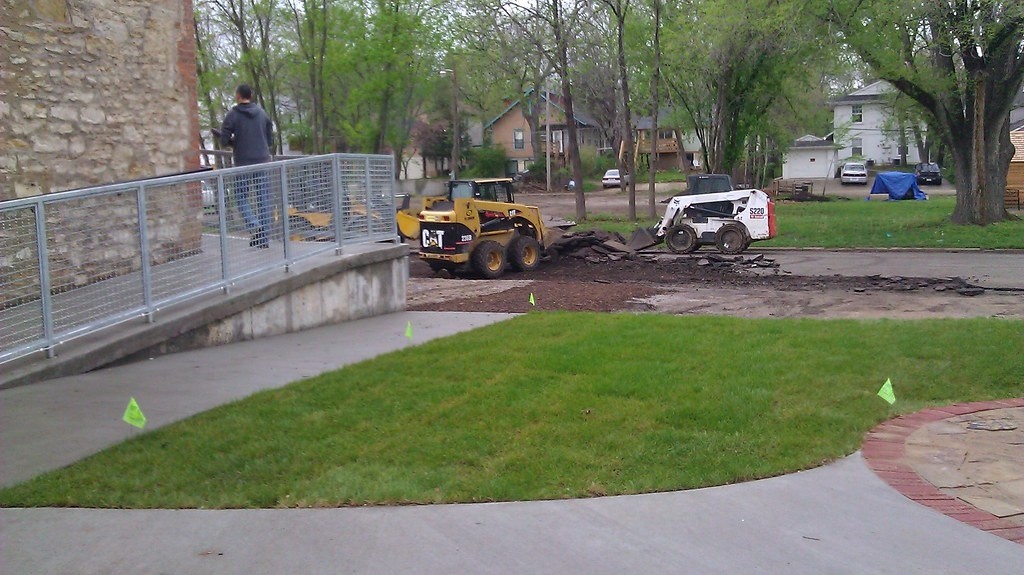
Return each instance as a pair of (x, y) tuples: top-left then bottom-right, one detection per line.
(249, 225), (269, 250)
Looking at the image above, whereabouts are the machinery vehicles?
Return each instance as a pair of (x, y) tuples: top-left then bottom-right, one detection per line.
(409, 171), (579, 280)
(627, 173), (778, 256)
(272, 180), (421, 243)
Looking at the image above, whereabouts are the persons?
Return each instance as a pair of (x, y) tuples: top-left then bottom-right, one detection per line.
(220, 85), (274, 248)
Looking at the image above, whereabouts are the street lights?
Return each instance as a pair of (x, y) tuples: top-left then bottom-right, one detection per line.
(438, 69), (460, 180)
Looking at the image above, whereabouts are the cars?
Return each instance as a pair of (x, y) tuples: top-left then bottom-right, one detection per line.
(912, 162), (944, 186)
(602, 168), (629, 188)
(840, 161), (869, 185)
